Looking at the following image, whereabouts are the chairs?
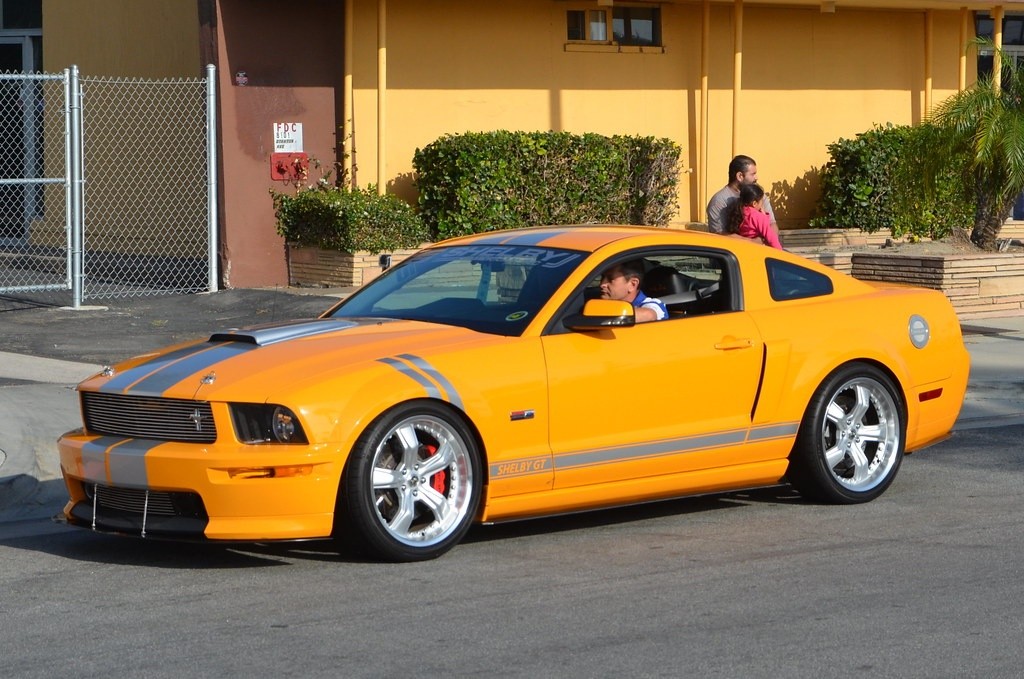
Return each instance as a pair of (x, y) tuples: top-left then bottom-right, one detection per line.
(642, 266), (694, 316)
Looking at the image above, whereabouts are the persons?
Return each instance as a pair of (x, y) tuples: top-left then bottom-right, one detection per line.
(707, 155), (782, 250)
(599, 257), (669, 323)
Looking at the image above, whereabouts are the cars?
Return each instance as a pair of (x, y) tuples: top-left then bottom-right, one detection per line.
(57, 223), (969, 565)
